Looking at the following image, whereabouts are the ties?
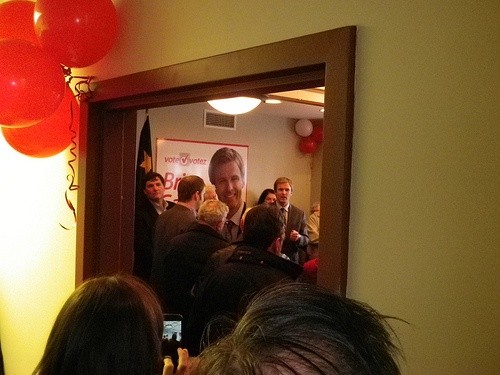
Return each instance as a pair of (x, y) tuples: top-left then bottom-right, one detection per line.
(281, 209), (289, 224)
(225, 222), (235, 242)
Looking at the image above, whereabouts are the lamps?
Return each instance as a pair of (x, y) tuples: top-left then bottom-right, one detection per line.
(207, 94), (268, 115)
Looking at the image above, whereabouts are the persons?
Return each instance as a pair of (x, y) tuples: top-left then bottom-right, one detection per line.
(189, 279), (410, 375)
(207, 147), (253, 242)
(135, 172), (320, 355)
(31, 274), (166, 375)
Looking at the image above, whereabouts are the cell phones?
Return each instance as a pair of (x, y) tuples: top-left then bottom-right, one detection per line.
(162, 313), (185, 370)
